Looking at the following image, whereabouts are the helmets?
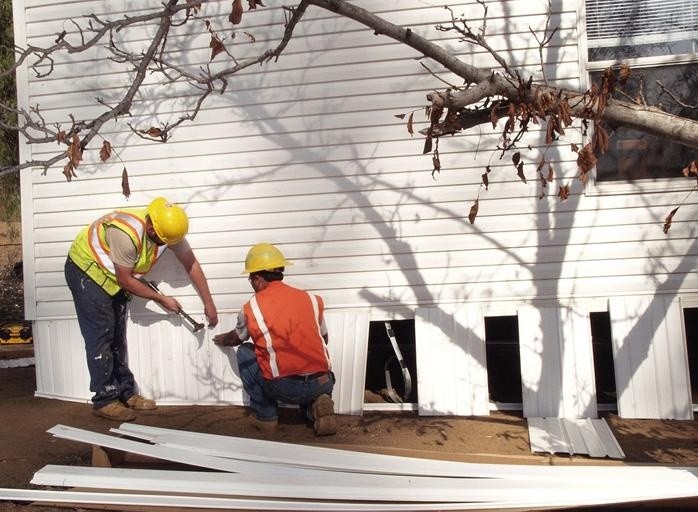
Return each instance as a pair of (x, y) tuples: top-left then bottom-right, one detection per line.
(148, 197), (189, 245)
(241, 243), (294, 275)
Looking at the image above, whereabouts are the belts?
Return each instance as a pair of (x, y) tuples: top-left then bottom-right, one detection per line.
(283, 372), (324, 381)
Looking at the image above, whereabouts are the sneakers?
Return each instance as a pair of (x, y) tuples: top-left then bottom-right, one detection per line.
(248, 413), (278, 431)
(311, 394), (337, 437)
(92, 402), (136, 421)
(127, 394), (157, 410)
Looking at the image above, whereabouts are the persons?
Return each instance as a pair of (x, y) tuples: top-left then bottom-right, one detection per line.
(64, 196), (218, 422)
(212, 242), (338, 438)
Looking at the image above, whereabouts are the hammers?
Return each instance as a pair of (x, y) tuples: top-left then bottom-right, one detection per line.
(147, 281), (205, 333)
